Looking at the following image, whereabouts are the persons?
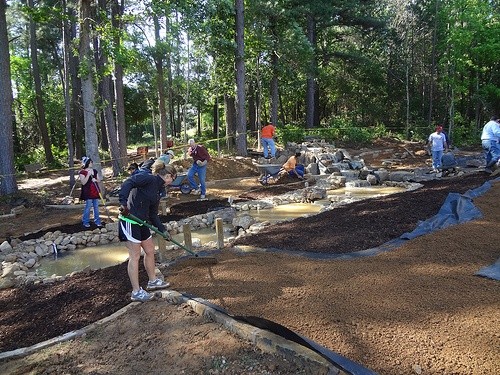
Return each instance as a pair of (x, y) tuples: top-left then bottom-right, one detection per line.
(427, 126), (447, 173)
(187, 139), (210, 199)
(261, 122), (275, 159)
(481, 116), (500, 170)
(283, 153), (304, 181)
(118, 164), (177, 302)
(78, 156), (103, 228)
(127, 150), (175, 176)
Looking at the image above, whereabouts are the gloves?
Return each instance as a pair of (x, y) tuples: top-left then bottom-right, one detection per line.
(120, 205), (130, 218)
(162, 229), (172, 242)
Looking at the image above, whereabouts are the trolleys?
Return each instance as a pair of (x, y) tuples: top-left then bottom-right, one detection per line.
(169, 171), (200, 195)
(256, 163), (285, 184)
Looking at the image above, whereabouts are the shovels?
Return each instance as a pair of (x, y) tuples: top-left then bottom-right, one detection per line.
(89, 171), (113, 222)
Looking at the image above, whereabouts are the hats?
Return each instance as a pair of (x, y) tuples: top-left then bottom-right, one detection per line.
(81, 156), (91, 168)
(167, 150), (175, 159)
(437, 127), (443, 132)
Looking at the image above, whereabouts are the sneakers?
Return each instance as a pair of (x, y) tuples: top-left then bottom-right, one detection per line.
(147, 278), (171, 289)
(131, 287), (157, 301)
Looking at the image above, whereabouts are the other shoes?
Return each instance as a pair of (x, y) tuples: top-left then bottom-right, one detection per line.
(83, 223), (91, 229)
(194, 188), (201, 195)
(200, 194), (206, 199)
(486, 167), (493, 174)
(94, 223), (103, 228)
